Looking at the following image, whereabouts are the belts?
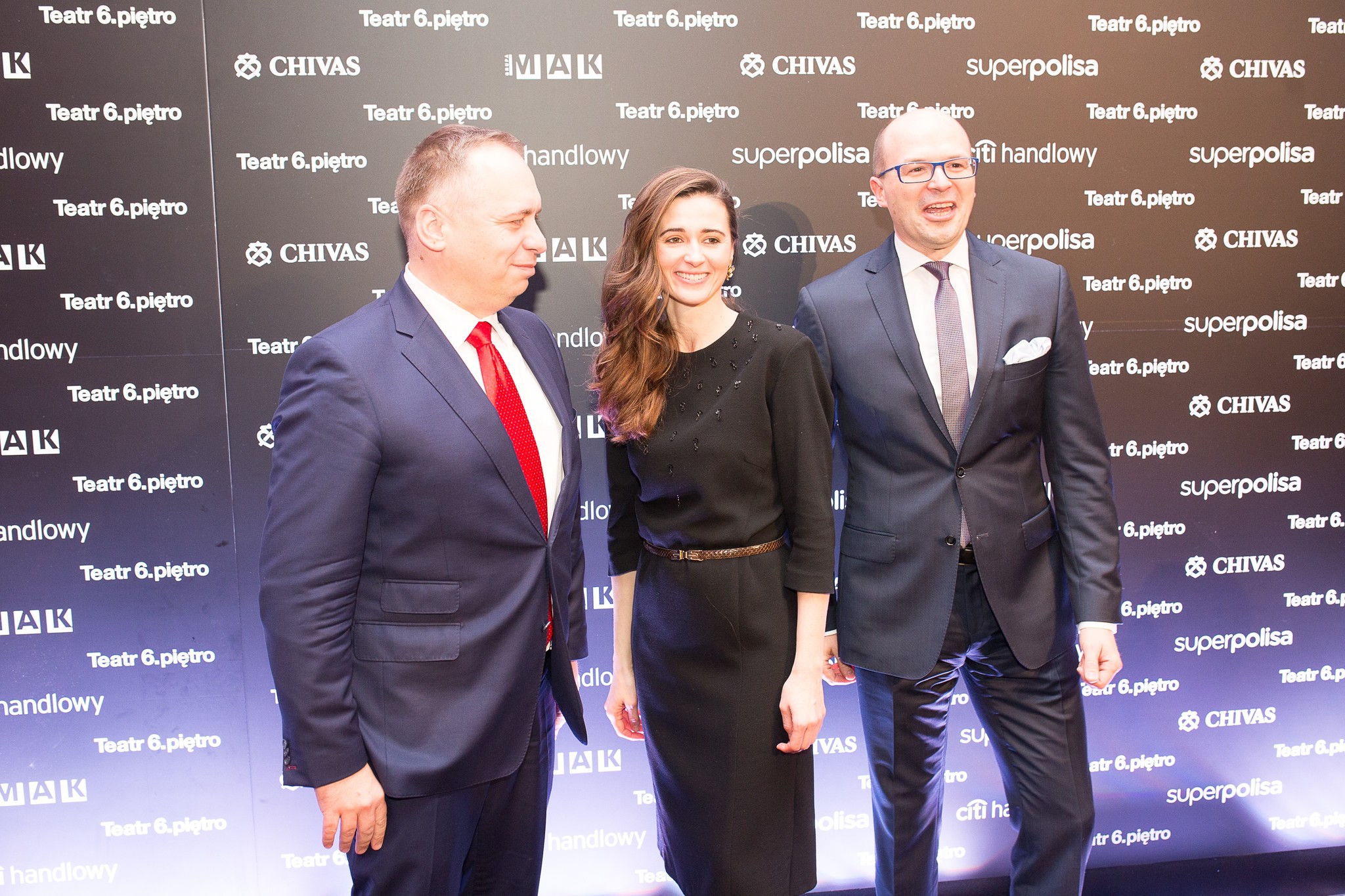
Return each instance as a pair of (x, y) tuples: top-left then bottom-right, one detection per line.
(958, 547), (978, 566)
(643, 537), (785, 562)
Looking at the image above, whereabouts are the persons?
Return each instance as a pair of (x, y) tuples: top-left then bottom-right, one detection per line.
(793, 107), (1125, 895)
(255, 126), (589, 896)
(586, 166), (836, 896)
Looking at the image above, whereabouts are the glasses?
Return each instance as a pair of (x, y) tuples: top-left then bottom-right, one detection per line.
(877, 156), (979, 183)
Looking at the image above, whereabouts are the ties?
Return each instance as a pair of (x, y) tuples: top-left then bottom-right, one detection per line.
(466, 322), (555, 646)
(923, 262), (970, 549)
